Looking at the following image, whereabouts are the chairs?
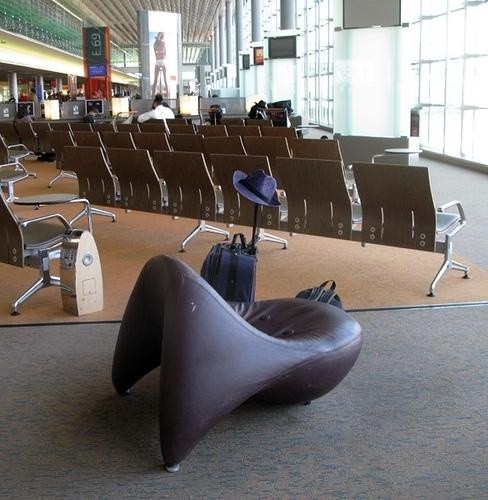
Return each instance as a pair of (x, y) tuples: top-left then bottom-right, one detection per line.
(111, 253), (363, 472)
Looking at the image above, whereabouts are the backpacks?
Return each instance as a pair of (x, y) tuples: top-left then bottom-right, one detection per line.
(295, 279), (343, 310)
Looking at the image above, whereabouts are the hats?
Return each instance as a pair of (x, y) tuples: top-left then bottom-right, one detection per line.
(232, 168), (283, 209)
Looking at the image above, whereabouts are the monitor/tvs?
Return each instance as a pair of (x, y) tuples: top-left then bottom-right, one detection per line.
(268, 36), (296, 58)
(343, 0), (401, 29)
(254, 47), (264, 65)
(16, 102), (35, 117)
(86, 99), (105, 115)
(243, 55), (250, 69)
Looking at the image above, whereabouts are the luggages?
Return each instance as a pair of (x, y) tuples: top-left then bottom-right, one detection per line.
(199, 232), (257, 303)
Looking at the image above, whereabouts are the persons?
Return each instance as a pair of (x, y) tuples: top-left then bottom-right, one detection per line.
(83, 107), (98, 123)
(137, 95), (176, 134)
(135, 93), (142, 99)
(151, 33), (168, 96)
(14, 107), (37, 135)
(48, 89), (83, 104)
(28, 88), (38, 104)
